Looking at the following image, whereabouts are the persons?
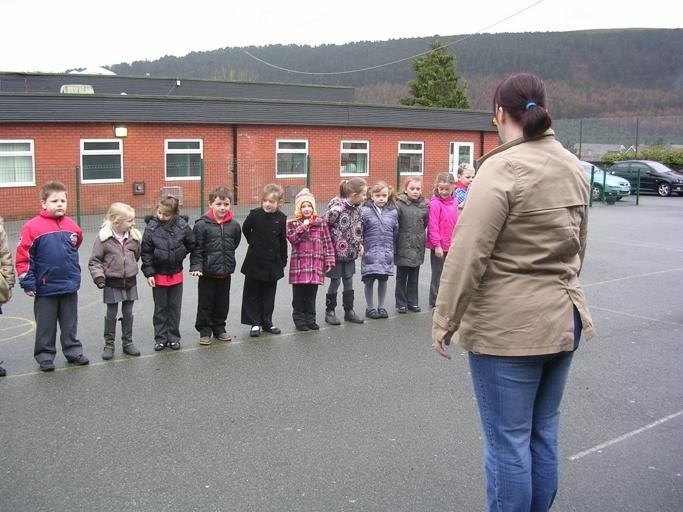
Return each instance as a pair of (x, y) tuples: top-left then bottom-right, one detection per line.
(394, 176), (429, 313)
(453, 163), (476, 209)
(0, 218), (15, 377)
(320, 177), (368, 325)
(424, 172), (460, 308)
(240, 184), (288, 337)
(360, 180), (400, 319)
(285, 187), (336, 331)
(15, 182), (89, 371)
(189, 186), (241, 345)
(431, 73), (597, 512)
(140, 196), (198, 351)
(88, 202), (143, 360)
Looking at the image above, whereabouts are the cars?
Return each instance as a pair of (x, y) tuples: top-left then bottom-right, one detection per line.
(576, 160), (631, 204)
(610, 160), (683, 196)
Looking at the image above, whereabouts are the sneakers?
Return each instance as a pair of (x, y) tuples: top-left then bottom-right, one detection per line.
(154, 343), (166, 350)
(0, 366), (6, 376)
(214, 333), (231, 341)
(263, 325), (281, 334)
(69, 354), (89, 365)
(398, 307), (408, 313)
(200, 335), (212, 345)
(308, 323), (319, 330)
(40, 360), (55, 371)
(378, 308), (388, 318)
(296, 324), (309, 331)
(167, 342), (180, 349)
(407, 305), (421, 312)
(250, 325), (260, 337)
(366, 307), (381, 319)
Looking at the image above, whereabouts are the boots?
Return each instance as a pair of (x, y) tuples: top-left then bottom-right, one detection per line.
(343, 290), (363, 323)
(119, 315), (141, 355)
(325, 292), (341, 325)
(102, 316), (117, 360)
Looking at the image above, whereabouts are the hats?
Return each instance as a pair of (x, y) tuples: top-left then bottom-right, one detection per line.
(295, 187), (317, 220)
